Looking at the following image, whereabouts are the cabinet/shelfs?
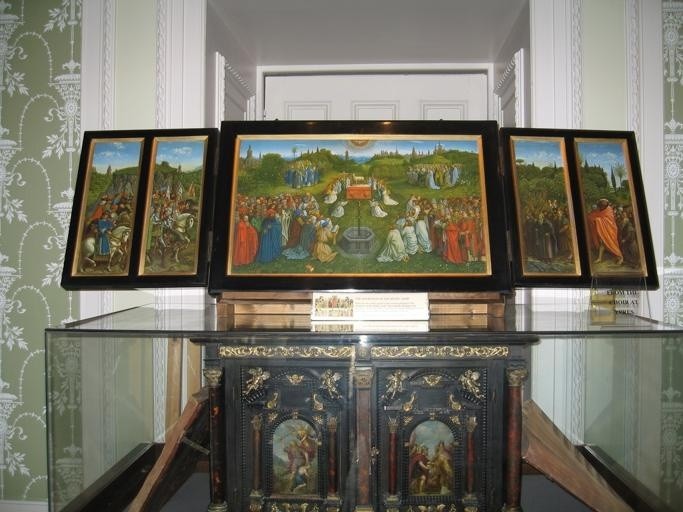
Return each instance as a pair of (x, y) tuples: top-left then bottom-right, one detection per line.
(40, 304), (682, 512)
(190, 335), (537, 509)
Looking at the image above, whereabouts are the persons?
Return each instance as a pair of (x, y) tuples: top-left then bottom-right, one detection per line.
(408, 435), (452, 495)
(285, 423), (313, 493)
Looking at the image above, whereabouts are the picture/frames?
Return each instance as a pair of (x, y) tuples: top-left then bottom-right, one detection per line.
(207, 118), (510, 297)
(60, 126), (219, 290)
(499, 126), (661, 291)
